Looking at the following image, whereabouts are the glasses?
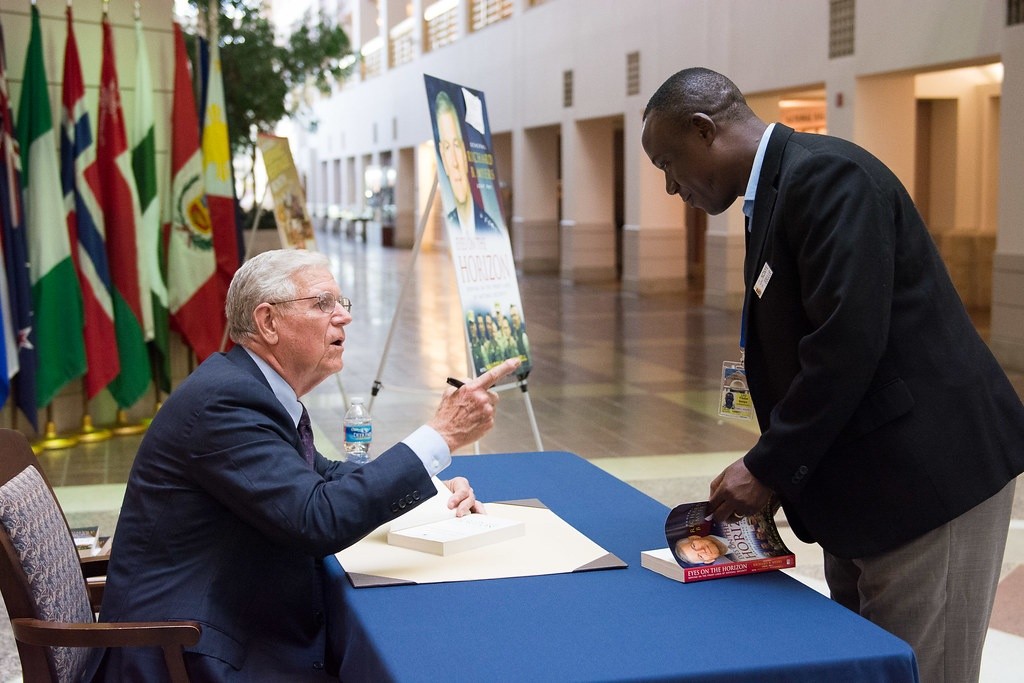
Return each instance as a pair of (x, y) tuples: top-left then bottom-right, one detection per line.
(269, 293), (352, 314)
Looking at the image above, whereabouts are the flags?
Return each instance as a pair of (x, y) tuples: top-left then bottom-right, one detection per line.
(0, 0), (241, 448)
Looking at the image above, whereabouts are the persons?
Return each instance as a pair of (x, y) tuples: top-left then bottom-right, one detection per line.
(434, 89), (502, 233)
(673, 529), (735, 566)
(640, 66), (1024, 683)
(723, 392), (734, 410)
(468, 301), (532, 370)
(82, 249), (521, 683)
(746, 510), (784, 559)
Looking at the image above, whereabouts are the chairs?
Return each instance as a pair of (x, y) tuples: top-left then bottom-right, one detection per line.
(0, 427), (203, 683)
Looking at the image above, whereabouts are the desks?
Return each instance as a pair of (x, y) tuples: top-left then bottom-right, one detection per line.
(306, 452), (919, 683)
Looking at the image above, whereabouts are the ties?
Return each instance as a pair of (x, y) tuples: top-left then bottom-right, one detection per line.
(297, 400), (315, 470)
(744, 214), (752, 287)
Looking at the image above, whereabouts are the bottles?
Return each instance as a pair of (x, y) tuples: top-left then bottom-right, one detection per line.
(342, 397), (373, 466)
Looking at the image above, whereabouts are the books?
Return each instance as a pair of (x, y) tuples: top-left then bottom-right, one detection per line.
(69, 527), (112, 559)
(387, 474), (522, 555)
(641, 487), (796, 583)
